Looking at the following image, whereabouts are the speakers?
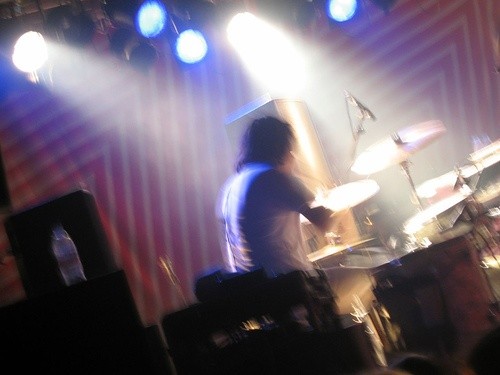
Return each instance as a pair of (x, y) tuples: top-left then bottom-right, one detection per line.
(4, 190), (152, 374)
(160, 269), (357, 375)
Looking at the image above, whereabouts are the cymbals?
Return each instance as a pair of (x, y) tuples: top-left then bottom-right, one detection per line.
(299, 179), (380, 225)
(308, 237), (376, 266)
(350, 125), (447, 174)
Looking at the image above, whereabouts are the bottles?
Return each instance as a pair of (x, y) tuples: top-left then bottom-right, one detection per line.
(50, 225), (88, 288)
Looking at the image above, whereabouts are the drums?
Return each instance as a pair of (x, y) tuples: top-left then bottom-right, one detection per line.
(372, 235), (496, 344)
(404, 193), (496, 253)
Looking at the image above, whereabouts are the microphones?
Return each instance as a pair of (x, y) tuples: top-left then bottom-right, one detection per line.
(346, 91), (375, 119)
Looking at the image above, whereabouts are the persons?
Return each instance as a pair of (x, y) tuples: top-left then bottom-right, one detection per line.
(217, 117), (360, 375)
